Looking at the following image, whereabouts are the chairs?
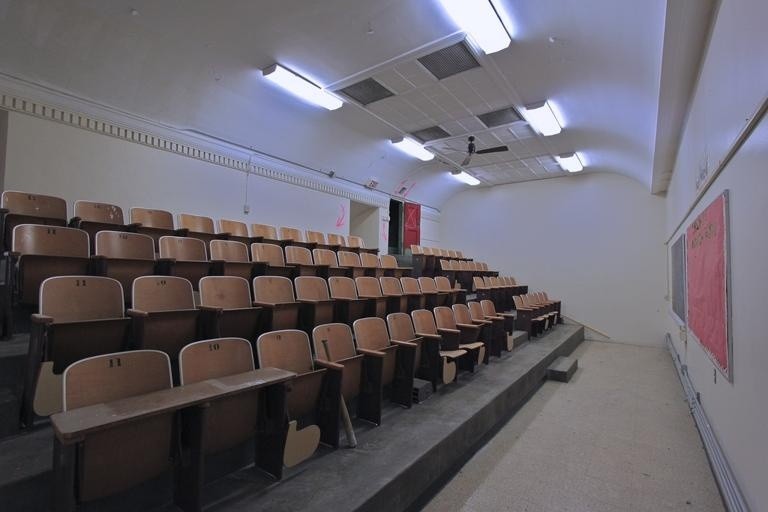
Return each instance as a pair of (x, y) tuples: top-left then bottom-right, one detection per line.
(512, 292), (561, 341)
(411, 245), (473, 279)
(257, 329), (326, 480)
(434, 259), (499, 295)
(179, 337), (256, 512)
(473, 276), (528, 312)
(51, 349), (173, 512)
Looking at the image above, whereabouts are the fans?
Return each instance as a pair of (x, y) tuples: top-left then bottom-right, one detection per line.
(442, 136), (508, 167)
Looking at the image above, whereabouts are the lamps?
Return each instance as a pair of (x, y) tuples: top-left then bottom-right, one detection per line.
(442, 0), (512, 55)
(261, 64), (343, 111)
(522, 99), (561, 137)
(391, 137), (434, 162)
(451, 170), (481, 186)
(554, 152), (585, 177)
